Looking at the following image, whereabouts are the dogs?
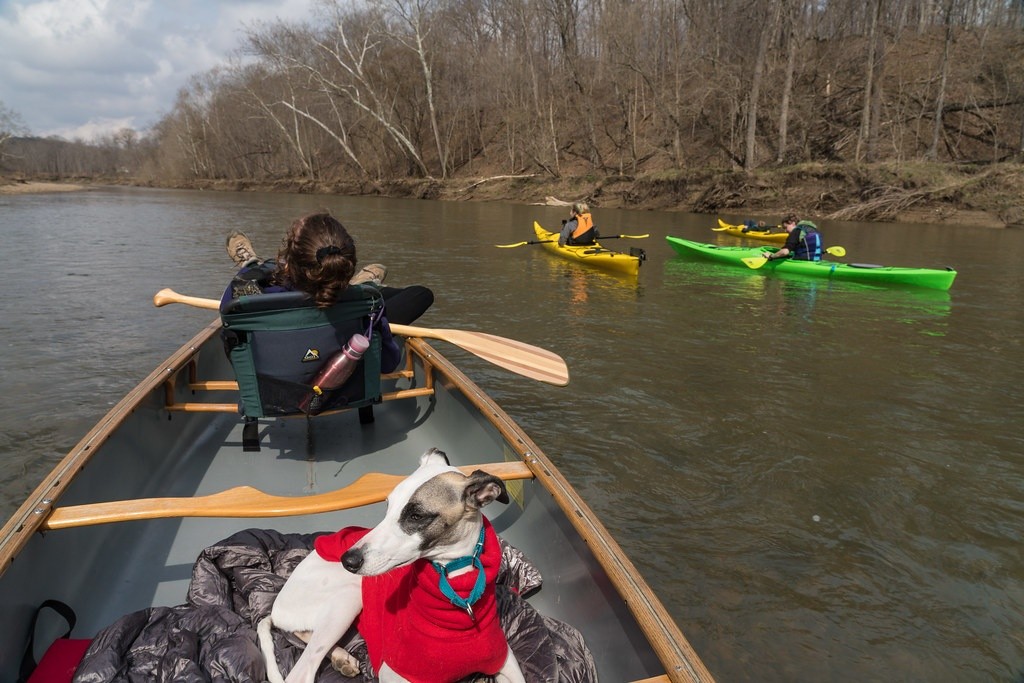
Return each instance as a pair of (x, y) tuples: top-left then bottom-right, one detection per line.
(257, 448), (524, 683)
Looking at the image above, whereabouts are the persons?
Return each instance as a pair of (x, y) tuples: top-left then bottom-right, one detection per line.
(558, 201), (600, 247)
(218, 211), (435, 375)
(762, 213), (822, 261)
(740, 219), (769, 234)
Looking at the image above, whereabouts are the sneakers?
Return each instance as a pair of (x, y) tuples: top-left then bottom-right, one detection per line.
(225, 228), (263, 269)
(349, 263), (388, 286)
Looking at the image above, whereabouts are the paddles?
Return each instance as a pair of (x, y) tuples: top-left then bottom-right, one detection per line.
(710, 224), (783, 232)
(153, 287), (570, 388)
(494, 233), (650, 248)
(740, 245), (846, 270)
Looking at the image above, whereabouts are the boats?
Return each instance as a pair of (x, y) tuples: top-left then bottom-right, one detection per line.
(717, 219), (789, 243)
(0, 315), (718, 683)
(533, 220), (648, 276)
(665, 235), (958, 292)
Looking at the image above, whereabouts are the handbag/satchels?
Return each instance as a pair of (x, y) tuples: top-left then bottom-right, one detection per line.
(16, 599), (91, 683)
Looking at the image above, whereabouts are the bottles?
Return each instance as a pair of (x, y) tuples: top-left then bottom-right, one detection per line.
(296, 334), (370, 417)
